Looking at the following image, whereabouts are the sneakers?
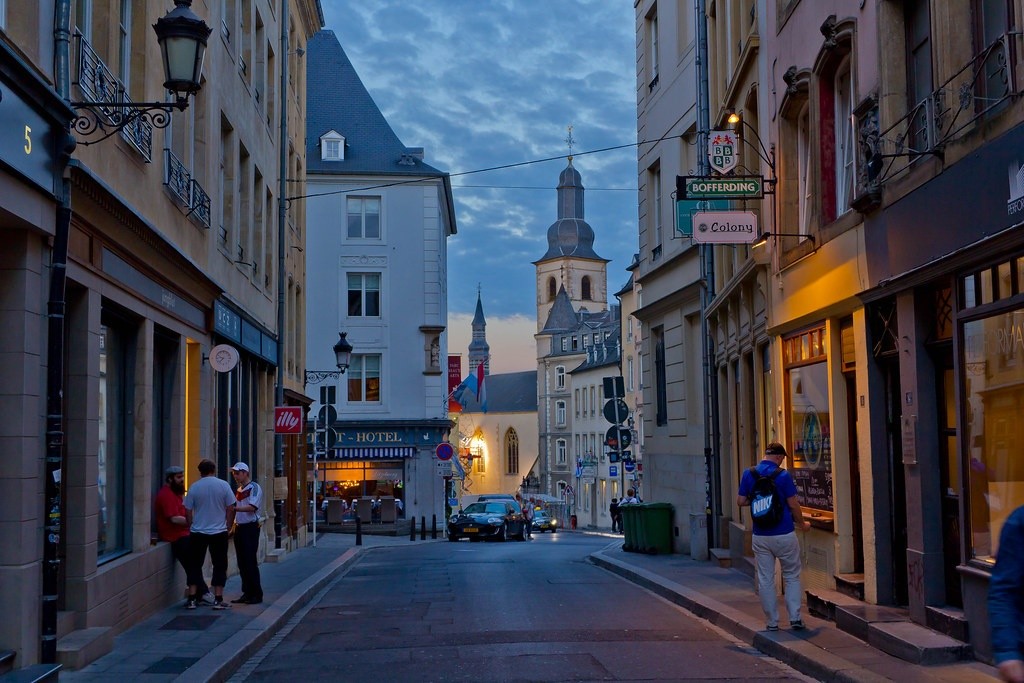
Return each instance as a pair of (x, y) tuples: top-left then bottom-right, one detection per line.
(790, 620), (805, 628)
(184, 601), (196, 608)
(768, 626), (778, 631)
(213, 600), (232, 608)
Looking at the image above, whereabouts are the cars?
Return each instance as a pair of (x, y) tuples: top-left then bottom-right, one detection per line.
(447, 494), (528, 541)
(531, 510), (557, 533)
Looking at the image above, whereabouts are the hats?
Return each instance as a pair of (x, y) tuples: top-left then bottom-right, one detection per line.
(765, 443), (790, 458)
(165, 466), (184, 475)
(230, 462), (249, 472)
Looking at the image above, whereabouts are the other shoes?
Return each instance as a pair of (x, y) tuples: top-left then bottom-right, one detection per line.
(245, 598), (261, 604)
(197, 592), (214, 605)
(231, 594), (248, 603)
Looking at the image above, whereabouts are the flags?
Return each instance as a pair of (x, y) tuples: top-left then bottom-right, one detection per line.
(453, 362), (487, 415)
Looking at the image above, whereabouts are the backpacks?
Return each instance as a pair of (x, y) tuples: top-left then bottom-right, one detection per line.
(750, 466), (786, 530)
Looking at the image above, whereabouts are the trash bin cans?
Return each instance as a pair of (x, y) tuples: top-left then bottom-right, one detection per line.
(617, 502), (644, 554)
(639, 502), (673, 554)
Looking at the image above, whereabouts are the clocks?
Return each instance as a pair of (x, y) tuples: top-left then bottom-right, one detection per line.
(208, 344), (240, 373)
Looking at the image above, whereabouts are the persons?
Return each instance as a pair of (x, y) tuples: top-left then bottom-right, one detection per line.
(515, 494), (534, 540)
(155, 466), (215, 605)
(608, 488), (636, 534)
(230, 462), (263, 604)
(986, 504), (1024, 683)
(183, 458), (236, 610)
(736, 443), (811, 631)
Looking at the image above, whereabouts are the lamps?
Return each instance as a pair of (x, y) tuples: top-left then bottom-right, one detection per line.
(66, 0), (213, 147)
(751, 231), (815, 250)
(302, 331), (355, 388)
(728, 106), (775, 175)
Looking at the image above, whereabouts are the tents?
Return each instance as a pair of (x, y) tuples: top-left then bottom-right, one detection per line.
(522, 493), (565, 504)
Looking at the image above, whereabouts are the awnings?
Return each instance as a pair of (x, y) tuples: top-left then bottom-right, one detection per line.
(307, 446), (418, 457)
